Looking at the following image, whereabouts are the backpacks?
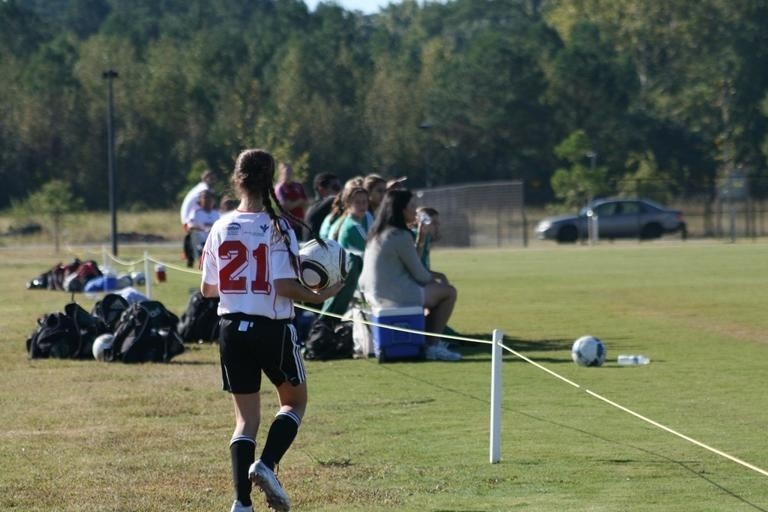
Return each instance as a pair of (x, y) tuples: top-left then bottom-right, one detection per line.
(24, 258), (220, 364)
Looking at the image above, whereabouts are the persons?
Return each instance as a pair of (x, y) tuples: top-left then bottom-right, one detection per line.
(199, 147), (346, 511)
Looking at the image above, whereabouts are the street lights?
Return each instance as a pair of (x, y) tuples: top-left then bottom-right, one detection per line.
(101, 69), (118, 257)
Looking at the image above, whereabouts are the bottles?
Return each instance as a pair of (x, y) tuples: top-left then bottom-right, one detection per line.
(416, 213), (431, 225)
(154, 264), (167, 283)
(617, 355), (651, 366)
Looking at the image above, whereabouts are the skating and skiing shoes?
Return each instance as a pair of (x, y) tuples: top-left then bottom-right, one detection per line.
(230, 499), (254, 511)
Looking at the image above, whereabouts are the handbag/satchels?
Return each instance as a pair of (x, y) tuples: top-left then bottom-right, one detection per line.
(301, 318), (355, 361)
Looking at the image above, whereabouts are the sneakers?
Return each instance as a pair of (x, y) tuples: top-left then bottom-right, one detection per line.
(248, 460), (290, 511)
(423, 340), (462, 362)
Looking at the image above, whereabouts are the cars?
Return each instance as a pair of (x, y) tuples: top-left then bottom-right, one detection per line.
(535, 198), (684, 243)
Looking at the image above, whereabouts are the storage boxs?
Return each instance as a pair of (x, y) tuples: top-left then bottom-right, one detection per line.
(372, 305), (425, 363)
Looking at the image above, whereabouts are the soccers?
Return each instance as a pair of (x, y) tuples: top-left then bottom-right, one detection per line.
(93, 333), (118, 363)
(116, 270), (146, 288)
(298, 238), (349, 291)
(571, 336), (606, 366)
